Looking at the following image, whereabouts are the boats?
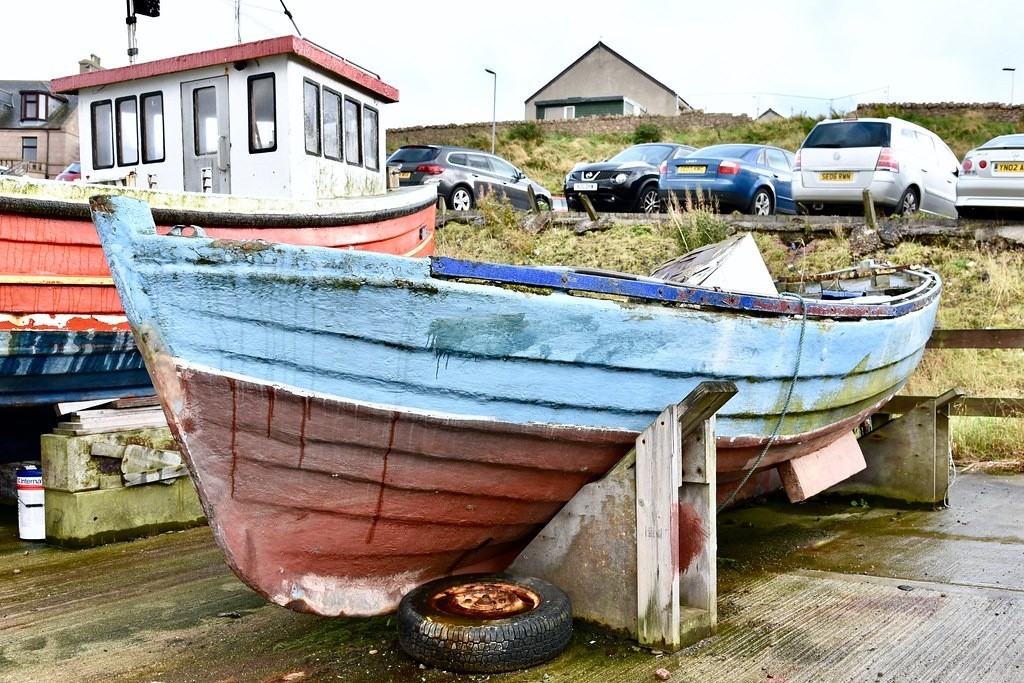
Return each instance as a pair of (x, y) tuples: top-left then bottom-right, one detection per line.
(1, 175), (446, 396)
(84, 190), (943, 619)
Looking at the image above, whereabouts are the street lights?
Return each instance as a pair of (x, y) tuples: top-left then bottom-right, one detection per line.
(484, 68), (497, 155)
(1001, 67), (1016, 105)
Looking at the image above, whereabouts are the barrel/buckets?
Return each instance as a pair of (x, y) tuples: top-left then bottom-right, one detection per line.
(12, 469), (46, 541)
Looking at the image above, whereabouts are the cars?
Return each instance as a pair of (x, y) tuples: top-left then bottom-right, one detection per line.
(657, 143), (796, 215)
(55, 160), (80, 181)
(550, 195), (568, 212)
(955, 131), (1024, 219)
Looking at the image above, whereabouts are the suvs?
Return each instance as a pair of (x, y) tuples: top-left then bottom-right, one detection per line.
(564, 143), (696, 214)
(790, 117), (963, 220)
(385, 144), (554, 214)
